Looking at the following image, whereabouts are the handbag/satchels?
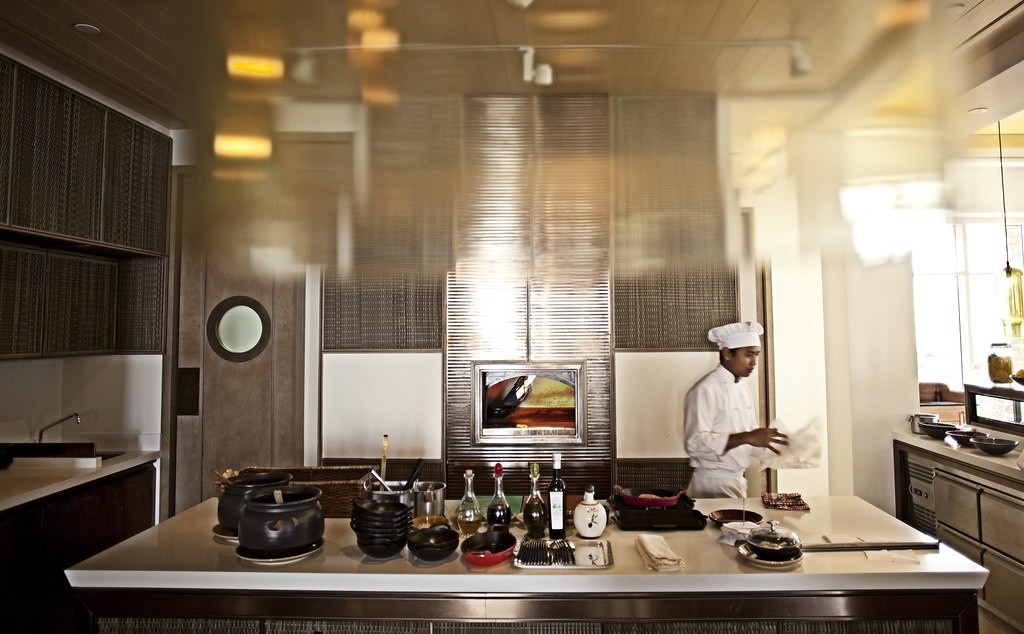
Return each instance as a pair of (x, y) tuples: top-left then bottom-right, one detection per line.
(757, 416), (824, 472)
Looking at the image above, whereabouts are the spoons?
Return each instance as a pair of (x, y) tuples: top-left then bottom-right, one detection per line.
(517, 539), (576, 566)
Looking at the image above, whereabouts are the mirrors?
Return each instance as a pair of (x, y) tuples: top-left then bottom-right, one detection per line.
(206, 295), (272, 364)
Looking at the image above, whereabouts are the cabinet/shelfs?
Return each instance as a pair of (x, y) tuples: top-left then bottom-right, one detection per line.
(0, 53), (173, 361)
(934, 461), (1024, 634)
(0, 460), (156, 634)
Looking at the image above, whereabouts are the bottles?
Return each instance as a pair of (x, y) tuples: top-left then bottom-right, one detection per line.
(486, 463), (511, 526)
(523, 463), (547, 539)
(455, 470), (482, 533)
(573, 485), (607, 538)
(988, 343), (1012, 383)
(548, 454), (566, 540)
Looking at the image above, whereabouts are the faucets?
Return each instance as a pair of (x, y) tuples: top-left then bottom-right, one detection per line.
(39, 412), (81, 443)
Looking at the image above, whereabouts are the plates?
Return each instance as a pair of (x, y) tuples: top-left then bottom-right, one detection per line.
(234, 537), (326, 565)
(737, 543), (803, 570)
(709, 509), (764, 524)
(212, 523), (240, 544)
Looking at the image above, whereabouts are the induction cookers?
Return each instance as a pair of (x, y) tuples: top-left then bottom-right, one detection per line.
(607, 495), (707, 530)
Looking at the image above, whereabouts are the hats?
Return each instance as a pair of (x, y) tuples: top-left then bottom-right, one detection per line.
(707, 320), (764, 350)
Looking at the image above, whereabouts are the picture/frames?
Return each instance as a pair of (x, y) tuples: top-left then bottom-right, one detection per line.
(469, 357), (588, 450)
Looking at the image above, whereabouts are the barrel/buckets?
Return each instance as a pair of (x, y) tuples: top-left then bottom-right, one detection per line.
(413, 482), (446, 519)
(364, 476), (419, 505)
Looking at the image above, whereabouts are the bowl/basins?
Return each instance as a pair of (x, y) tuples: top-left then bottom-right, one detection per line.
(1009, 374), (1024, 386)
(746, 519), (803, 559)
(910, 413), (1019, 455)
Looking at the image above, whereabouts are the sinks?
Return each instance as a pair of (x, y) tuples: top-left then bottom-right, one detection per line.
(96, 450), (125, 461)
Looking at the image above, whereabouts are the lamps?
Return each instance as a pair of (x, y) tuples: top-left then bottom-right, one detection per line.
(998, 120), (1024, 337)
(791, 39), (814, 76)
(518, 44), (553, 87)
(287, 55), (314, 84)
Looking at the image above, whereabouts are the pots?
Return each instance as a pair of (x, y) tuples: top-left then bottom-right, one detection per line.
(461, 524), (517, 566)
(217, 473), (293, 534)
(349, 496), (459, 562)
(237, 487), (325, 555)
(615, 484), (686, 506)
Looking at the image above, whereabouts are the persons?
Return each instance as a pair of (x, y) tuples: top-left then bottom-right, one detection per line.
(682, 321), (789, 498)
